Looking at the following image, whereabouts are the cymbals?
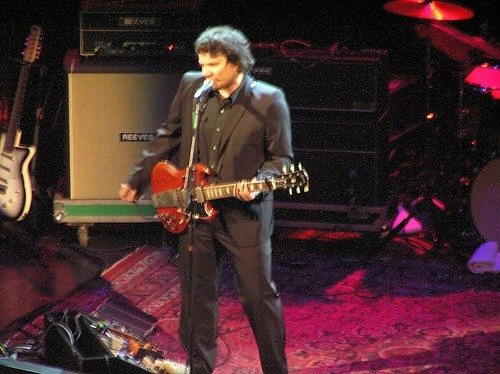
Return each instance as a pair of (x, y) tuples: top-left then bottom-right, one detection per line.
(431, 22), (500, 62)
(384, 0), (473, 21)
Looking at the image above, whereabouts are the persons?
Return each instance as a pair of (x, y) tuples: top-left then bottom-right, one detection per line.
(120, 26), (296, 374)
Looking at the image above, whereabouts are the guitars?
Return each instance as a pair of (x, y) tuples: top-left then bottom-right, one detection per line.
(151, 160), (310, 234)
(0, 22), (42, 221)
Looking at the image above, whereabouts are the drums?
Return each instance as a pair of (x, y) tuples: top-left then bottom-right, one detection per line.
(457, 156), (500, 245)
(447, 60), (500, 103)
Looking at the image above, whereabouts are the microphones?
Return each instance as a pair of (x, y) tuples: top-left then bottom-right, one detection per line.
(193, 78), (214, 100)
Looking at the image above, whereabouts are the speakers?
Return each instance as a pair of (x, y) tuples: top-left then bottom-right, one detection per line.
(67, 60), (185, 201)
